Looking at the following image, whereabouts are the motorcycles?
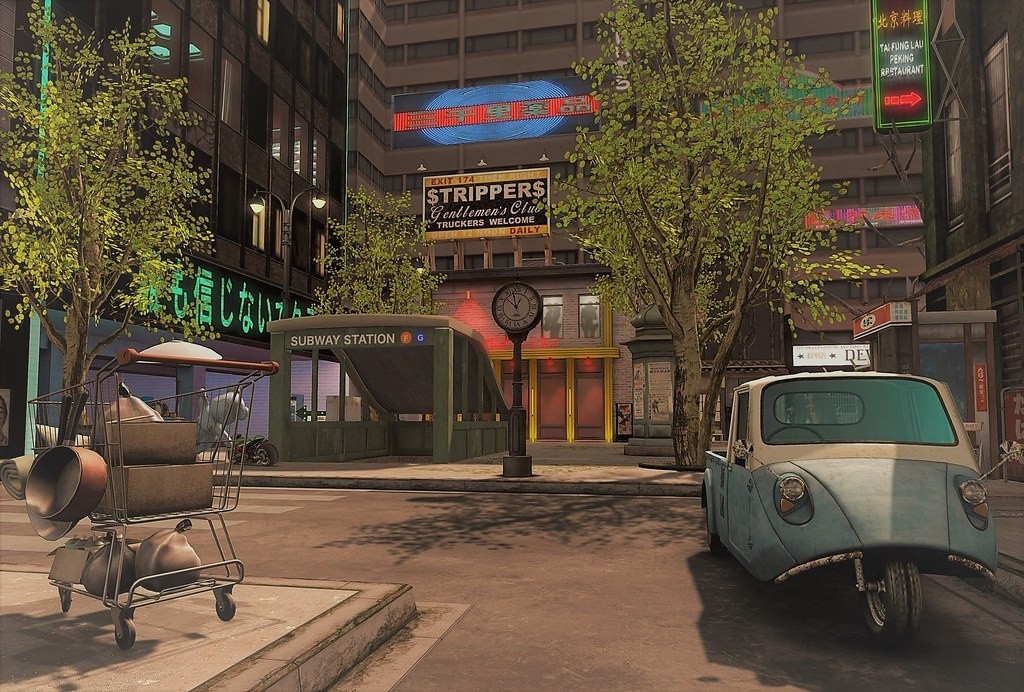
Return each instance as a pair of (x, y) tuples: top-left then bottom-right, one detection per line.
(228, 432), (280, 466)
(699, 367), (997, 651)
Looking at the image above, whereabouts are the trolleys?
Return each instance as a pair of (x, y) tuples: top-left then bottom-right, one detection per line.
(25, 348), (280, 650)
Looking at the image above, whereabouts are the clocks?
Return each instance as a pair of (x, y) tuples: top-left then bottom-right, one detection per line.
(491, 282), (542, 332)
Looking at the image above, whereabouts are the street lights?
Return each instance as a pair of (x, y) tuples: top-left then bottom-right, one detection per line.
(250, 183), (326, 319)
